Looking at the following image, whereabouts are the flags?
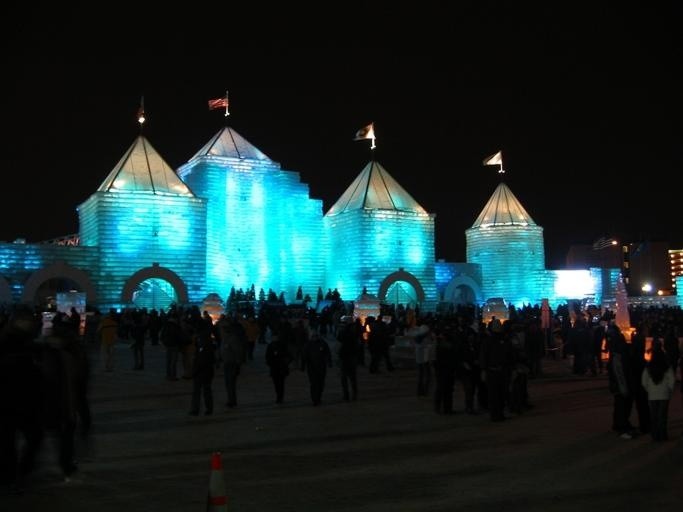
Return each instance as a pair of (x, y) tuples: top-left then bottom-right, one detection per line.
(353, 123), (375, 141)
(207, 95), (229, 110)
(482, 151), (502, 167)
(136, 96), (144, 121)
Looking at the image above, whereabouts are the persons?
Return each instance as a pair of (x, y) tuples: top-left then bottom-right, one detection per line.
(0, 287), (683, 510)
